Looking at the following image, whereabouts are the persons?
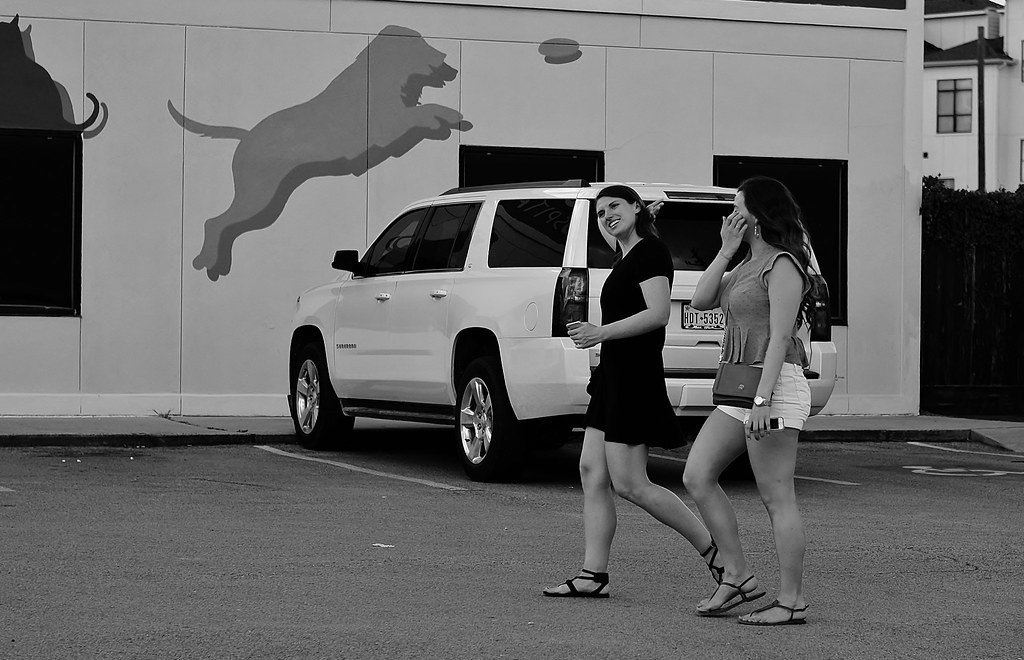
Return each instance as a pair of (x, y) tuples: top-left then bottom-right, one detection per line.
(682, 177), (820, 625)
(543, 185), (725, 598)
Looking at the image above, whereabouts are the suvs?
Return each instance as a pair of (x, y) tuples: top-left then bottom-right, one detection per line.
(288, 179), (839, 489)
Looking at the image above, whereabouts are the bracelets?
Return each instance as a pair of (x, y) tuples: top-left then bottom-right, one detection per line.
(719, 250), (732, 260)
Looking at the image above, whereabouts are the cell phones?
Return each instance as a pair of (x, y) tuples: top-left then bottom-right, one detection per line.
(566, 321), (582, 345)
(745, 417), (785, 433)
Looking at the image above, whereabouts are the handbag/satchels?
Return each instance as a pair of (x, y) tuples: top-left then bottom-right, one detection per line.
(712, 363), (774, 409)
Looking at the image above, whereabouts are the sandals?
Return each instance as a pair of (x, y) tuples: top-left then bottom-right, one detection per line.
(737, 599), (809, 626)
(700, 534), (725, 585)
(698, 575), (766, 615)
(542, 569), (610, 598)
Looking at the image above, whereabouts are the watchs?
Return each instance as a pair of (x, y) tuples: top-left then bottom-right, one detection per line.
(753, 396), (772, 407)
